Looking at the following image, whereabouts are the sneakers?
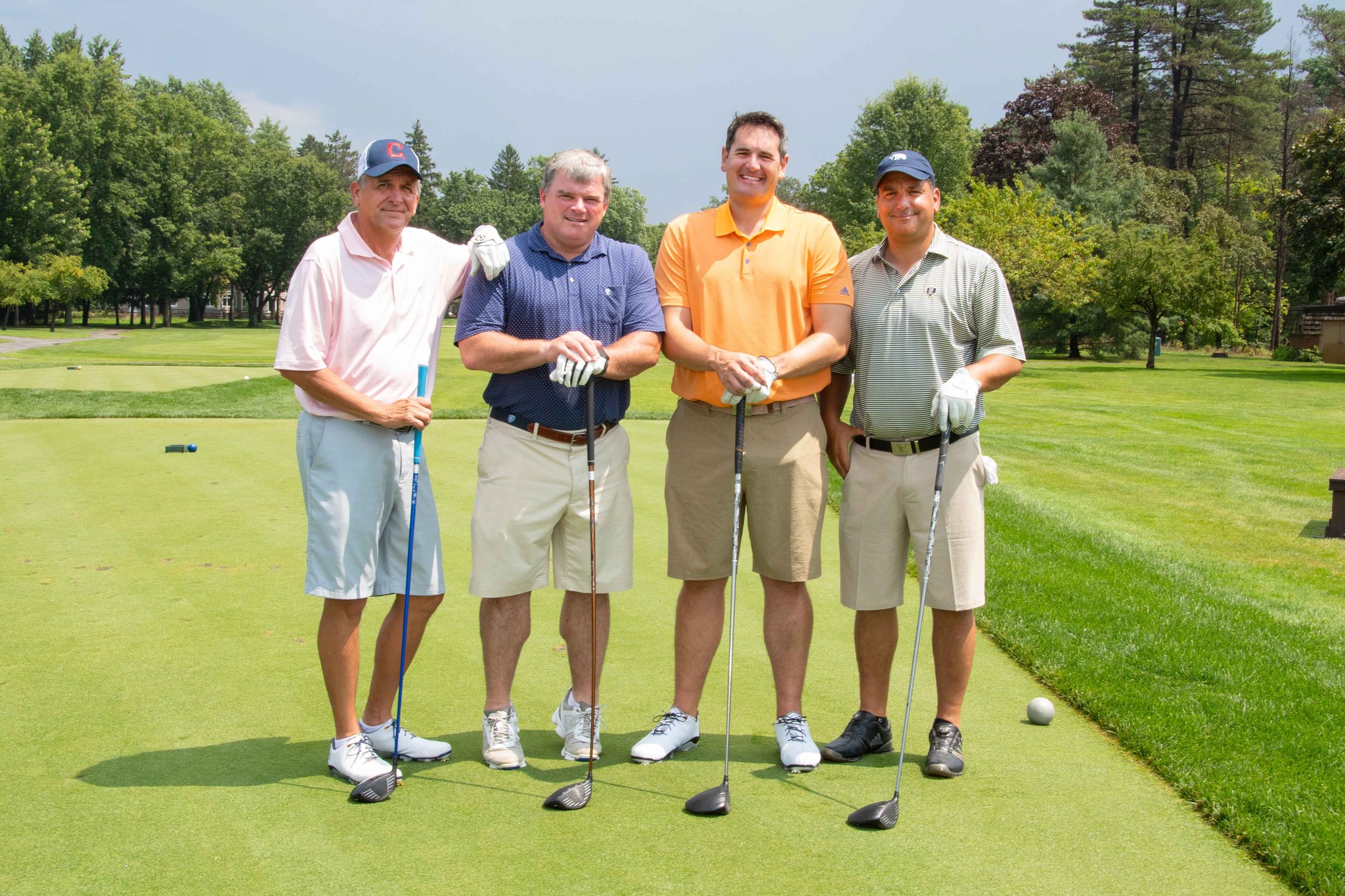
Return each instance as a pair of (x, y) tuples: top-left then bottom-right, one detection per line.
(819, 711), (894, 762)
(924, 723), (964, 777)
(630, 706), (701, 765)
(772, 711), (822, 773)
(327, 727), (403, 787)
(367, 719), (452, 762)
(551, 686), (607, 762)
(482, 699), (527, 770)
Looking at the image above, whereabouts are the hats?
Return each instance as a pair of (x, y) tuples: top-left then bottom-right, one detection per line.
(873, 150), (935, 187)
(357, 138), (424, 181)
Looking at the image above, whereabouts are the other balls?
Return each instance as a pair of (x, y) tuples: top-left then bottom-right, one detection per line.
(244, 375), (249, 379)
(1027, 697), (1057, 726)
(188, 444), (198, 452)
(77, 366), (82, 370)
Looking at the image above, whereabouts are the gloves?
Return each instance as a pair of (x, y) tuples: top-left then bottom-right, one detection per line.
(549, 348), (609, 387)
(468, 225), (510, 281)
(930, 368), (982, 432)
(720, 355), (778, 406)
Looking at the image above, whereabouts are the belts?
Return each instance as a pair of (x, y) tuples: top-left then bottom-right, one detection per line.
(852, 422), (979, 456)
(490, 406), (619, 446)
(680, 393), (816, 415)
(370, 421), (416, 432)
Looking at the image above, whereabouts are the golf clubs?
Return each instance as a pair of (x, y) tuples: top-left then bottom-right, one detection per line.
(543, 377), (597, 812)
(349, 362), (432, 803)
(682, 393), (746, 817)
(848, 418), (953, 830)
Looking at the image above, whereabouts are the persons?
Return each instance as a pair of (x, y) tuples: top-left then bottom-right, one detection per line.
(653, 112), (855, 773)
(274, 138), (511, 786)
(815, 150), (1027, 778)
(452, 148), (665, 771)
